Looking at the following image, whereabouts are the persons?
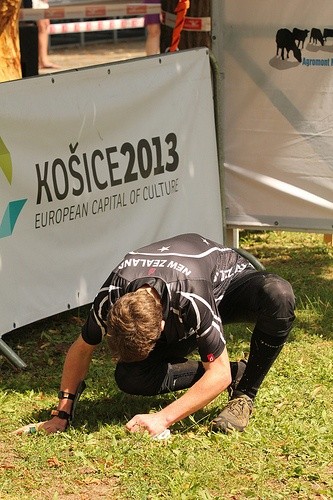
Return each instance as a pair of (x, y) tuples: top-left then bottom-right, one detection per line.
(0, 0), (211, 83)
(12, 233), (296, 439)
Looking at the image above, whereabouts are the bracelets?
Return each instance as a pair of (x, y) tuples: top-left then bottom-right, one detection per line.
(50, 408), (69, 421)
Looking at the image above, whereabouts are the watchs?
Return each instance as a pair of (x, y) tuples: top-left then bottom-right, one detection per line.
(56, 390), (76, 401)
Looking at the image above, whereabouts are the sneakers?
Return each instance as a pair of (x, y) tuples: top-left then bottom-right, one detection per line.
(209, 389), (254, 434)
(227, 359), (247, 398)
